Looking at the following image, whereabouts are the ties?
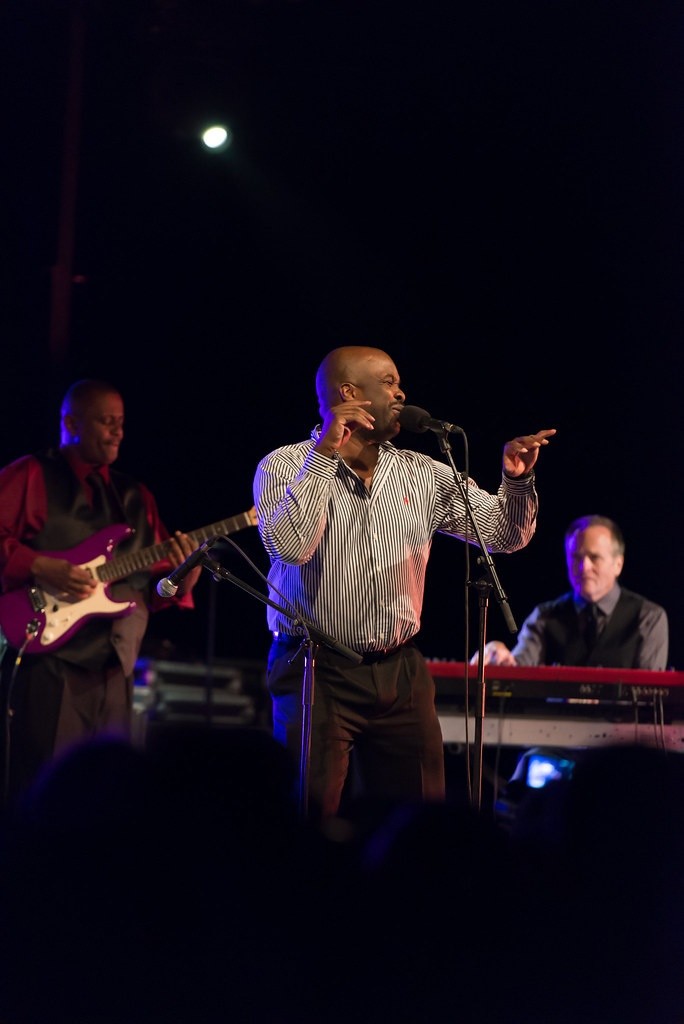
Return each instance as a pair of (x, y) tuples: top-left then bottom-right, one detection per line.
(583, 603), (602, 649)
(85, 471), (114, 530)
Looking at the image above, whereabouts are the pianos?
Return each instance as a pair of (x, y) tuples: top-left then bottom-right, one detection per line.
(405, 651), (684, 723)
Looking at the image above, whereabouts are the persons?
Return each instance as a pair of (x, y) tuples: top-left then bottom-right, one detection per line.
(254, 350), (558, 814)
(0, 381), (200, 756)
(0, 734), (684, 1024)
(469, 514), (670, 672)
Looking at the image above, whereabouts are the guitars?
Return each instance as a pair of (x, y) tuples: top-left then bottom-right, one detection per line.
(0, 493), (292, 666)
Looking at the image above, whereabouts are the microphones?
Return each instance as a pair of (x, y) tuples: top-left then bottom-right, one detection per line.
(157, 536), (220, 597)
(400, 405), (463, 434)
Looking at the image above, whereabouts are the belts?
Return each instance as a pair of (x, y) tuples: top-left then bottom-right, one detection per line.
(273, 631), (409, 664)
